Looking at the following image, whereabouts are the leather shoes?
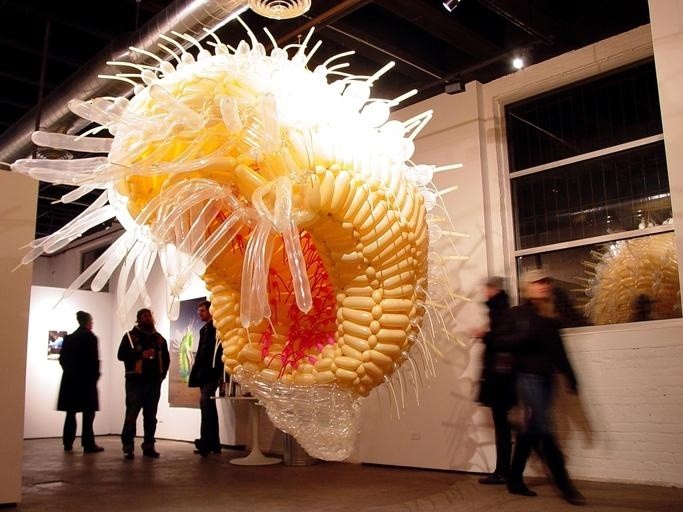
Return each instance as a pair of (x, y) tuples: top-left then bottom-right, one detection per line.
(143, 447), (160, 458)
(84, 445), (104, 454)
(63, 443), (73, 451)
(562, 488), (587, 506)
(478, 473), (538, 497)
(123, 450), (134, 459)
(194, 439), (222, 456)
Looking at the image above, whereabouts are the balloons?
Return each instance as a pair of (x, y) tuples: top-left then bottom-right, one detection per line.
(0, 19), (472, 459)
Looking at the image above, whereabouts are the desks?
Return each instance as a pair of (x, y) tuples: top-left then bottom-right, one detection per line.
(210, 396), (282, 466)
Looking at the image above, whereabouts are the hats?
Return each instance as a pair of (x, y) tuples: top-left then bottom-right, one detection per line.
(521, 268), (552, 283)
(76, 311), (93, 324)
(484, 276), (504, 287)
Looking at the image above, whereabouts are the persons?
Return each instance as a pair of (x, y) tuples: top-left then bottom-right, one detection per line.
(57, 311), (105, 454)
(497, 270), (587, 505)
(188, 302), (230, 457)
(467, 276), (517, 484)
(48, 333), (64, 354)
(118, 309), (170, 458)
